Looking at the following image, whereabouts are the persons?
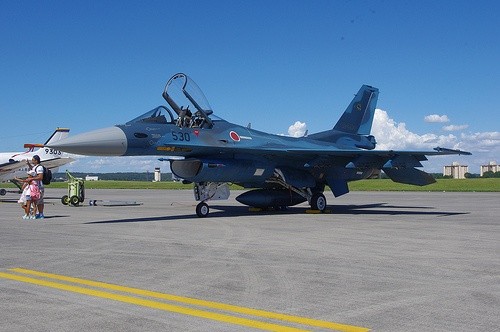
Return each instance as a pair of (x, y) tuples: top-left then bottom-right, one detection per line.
(26, 154), (45, 220)
(14, 170), (37, 220)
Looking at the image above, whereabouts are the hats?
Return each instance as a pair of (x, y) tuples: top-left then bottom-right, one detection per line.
(33, 154), (40, 162)
(25, 170), (37, 177)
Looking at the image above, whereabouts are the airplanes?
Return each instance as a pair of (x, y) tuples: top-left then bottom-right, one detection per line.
(0, 128), (76, 197)
(47, 72), (473, 218)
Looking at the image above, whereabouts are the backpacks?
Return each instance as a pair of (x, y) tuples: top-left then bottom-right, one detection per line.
(35, 164), (52, 185)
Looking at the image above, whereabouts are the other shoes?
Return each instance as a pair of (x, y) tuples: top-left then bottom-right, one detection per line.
(21, 213), (35, 220)
(35, 213), (45, 220)
